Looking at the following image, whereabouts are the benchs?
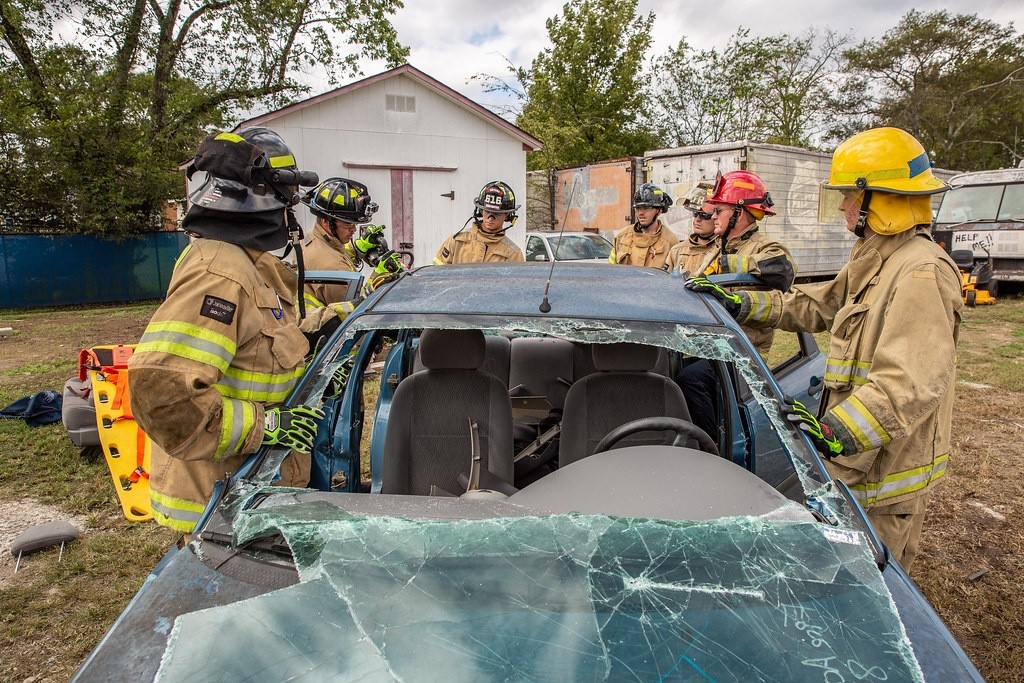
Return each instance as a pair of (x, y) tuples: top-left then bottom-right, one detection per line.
(408, 335), (683, 427)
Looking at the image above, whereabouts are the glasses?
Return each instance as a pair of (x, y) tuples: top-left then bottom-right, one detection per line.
(713, 207), (736, 217)
(692, 209), (713, 220)
(480, 208), (510, 221)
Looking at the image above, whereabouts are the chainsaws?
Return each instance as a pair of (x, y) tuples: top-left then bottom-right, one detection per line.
(356, 224), (407, 276)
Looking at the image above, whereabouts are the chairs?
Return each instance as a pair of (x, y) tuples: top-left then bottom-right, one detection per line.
(559, 343), (699, 469)
(380, 328), (514, 498)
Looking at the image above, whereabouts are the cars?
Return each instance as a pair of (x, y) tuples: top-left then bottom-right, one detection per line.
(70, 261), (984, 683)
(526, 230), (615, 264)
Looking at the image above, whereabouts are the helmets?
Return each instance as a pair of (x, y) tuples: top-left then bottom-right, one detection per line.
(189, 125), (298, 212)
(705, 170), (777, 218)
(684, 181), (716, 214)
(822, 126), (952, 196)
(299, 177), (372, 223)
(632, 183), (669, 208)
(474, 181), (517, 212)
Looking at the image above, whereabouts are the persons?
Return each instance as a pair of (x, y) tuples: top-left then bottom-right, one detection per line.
(295, 176), (404, 334)
(697, 170), (798, 366)
(608, 182), (680, 271)
(684, 127), (963, 576)
(665, 182), (722, 280)
(432, 181), (524, 267)
(127, 127), (398, 541)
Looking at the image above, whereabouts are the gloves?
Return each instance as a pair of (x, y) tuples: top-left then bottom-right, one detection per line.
(362, 272), (399, 300)
(682, 275), (741, 320)
(259, 404), (326, 455)
(364, 250), (402, 289)
(305, 335), (354, 399)
(354, 224), (387, 253)
(698, 257), (724, 278)
(778, 394), (843, 460)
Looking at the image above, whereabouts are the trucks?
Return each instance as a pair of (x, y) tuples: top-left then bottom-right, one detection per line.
(931, 165), (1024, 282)
